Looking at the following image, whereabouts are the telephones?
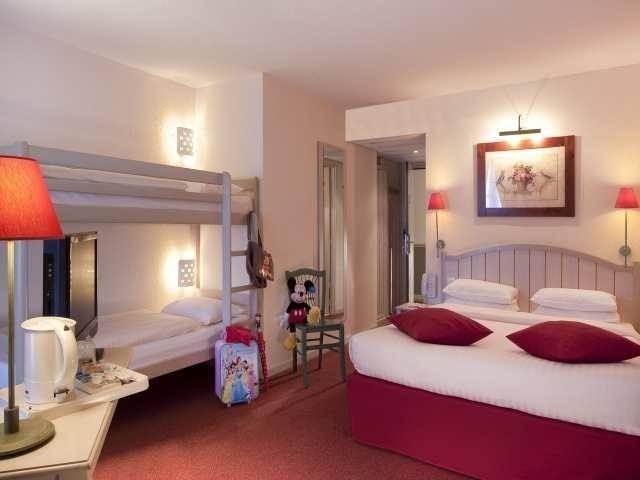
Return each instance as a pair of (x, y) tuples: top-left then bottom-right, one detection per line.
(421, 272), (438, 299)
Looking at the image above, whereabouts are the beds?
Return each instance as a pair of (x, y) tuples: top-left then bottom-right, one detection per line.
(347, 244), (639, 480)
(0, 139), (261, 388)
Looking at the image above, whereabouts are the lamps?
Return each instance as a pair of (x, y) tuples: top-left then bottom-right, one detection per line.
(0, 155), (64, 458)
(428, 193), (447, 248)
(614, 185), (639, 257)
(177, 127), (194, 156)
(178, 259), (196, 287)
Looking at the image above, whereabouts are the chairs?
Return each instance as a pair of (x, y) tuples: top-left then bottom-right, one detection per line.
(285, 267), (346, 388)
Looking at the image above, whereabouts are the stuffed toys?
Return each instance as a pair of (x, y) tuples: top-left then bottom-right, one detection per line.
(276, 277), (321, 351)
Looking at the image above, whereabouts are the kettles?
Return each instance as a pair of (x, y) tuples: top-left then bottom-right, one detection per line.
(20, 316), (78, 405)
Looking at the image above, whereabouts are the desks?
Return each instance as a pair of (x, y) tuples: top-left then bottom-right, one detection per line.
(0, 346), (134, 480)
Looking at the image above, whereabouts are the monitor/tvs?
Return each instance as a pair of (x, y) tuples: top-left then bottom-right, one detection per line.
(41, 231), (105, 361)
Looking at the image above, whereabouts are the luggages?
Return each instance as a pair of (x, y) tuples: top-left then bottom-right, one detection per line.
(214, 339), (260, 408)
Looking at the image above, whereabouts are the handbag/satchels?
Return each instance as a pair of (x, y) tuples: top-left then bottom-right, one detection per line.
(244, 211), (276, 289)
(225, 325), (252, 346)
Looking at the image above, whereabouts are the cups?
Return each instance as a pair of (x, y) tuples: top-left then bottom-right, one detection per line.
(77, 340), (97, 379)
(89, 372), (104, 384)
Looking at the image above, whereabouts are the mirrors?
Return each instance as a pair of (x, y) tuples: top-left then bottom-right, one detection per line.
(319, 140), (346, 321)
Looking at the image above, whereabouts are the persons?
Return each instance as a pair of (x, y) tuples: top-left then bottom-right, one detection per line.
(222, 356), (255, 402)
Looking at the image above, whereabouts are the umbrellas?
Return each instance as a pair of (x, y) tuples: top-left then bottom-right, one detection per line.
(255, 312), (269, 391)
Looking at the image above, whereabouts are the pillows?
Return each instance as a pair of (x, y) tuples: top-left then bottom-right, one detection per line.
(529, 287), (617, 313)
(443, 298), (519, 312)
(182, 179), (244, 195)
(387, 307), (493, 344)
(161, 295), (246, 325)
(504, 320), (639, 364)
(441, 278), (520, 305)
(532, 306), (621, 323)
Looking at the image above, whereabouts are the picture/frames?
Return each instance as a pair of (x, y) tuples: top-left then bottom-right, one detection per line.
(476, 136), (575, 217)
